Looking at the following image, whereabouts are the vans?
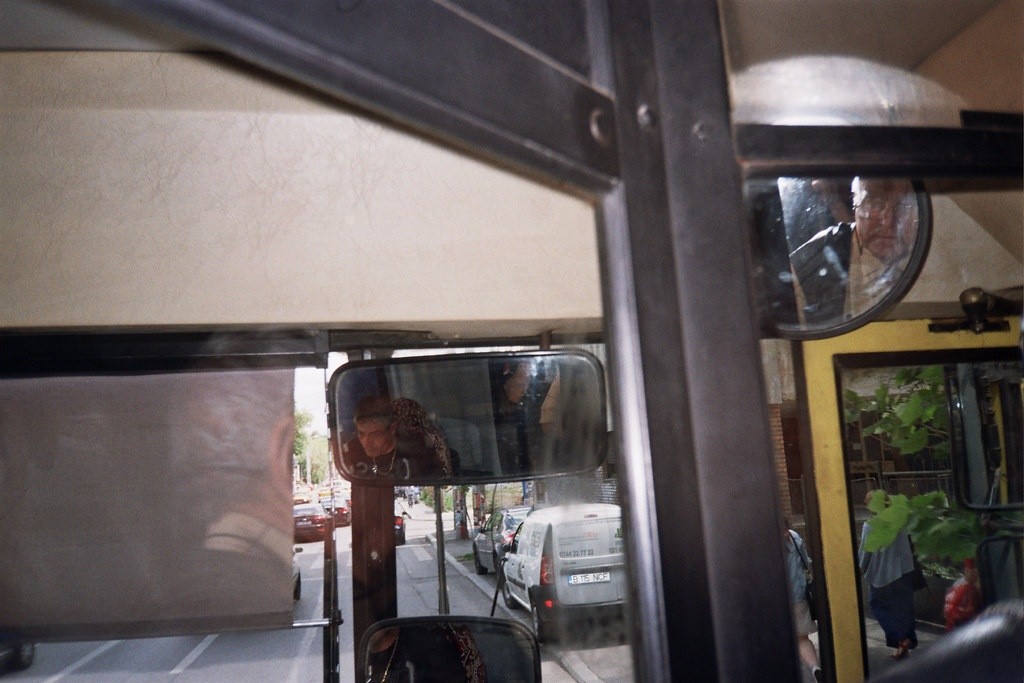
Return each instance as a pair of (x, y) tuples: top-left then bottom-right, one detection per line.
(501, 504), (627, 645)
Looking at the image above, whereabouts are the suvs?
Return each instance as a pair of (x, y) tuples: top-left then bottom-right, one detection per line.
(472, 505), (532, 589)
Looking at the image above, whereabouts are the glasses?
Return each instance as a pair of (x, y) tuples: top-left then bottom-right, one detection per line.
(852, 202), (917, 219)
(357, 418), (393, 440)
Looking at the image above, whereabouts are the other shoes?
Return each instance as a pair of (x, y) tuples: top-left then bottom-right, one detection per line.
(812, 665), (822, 683)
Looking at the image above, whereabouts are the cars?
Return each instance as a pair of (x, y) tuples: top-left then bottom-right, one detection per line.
(393, 485), (408, 546)
(322, 498), (351, 527)
(294, 504), (332, 542)
(293, 476), (353, 507)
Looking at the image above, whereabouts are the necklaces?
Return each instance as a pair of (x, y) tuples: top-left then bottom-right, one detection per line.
(372, 445), (397, 475)
(365, 627), (399, 683)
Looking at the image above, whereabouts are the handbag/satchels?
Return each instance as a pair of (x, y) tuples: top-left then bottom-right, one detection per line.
(908, 534), (926, 591)
(805, 582), (820, 621)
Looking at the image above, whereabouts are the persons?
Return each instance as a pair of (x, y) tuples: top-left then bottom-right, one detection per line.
(857, 489), (918, 661)
(343, 394), (432, 482)
(779, 515), (824, 683)
(363, 623), (467, 683)
(490, 356), (569, 478)
(788, 176), (920, 331)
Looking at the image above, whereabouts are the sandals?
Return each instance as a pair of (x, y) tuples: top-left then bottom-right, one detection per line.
(888, 638), (911, 658)
